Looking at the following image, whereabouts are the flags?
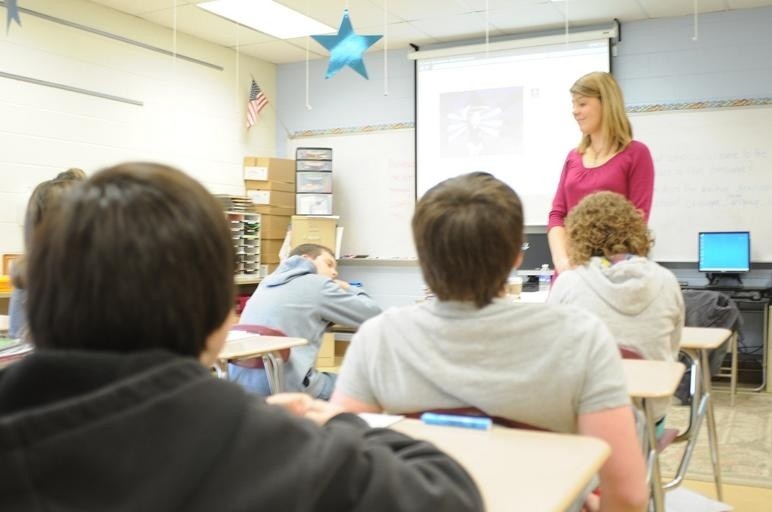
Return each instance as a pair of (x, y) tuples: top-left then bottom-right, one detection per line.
(246, 80), (269, 129)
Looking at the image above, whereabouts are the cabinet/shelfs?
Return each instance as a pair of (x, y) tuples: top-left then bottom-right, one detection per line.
(224, 210), (262, 277)
(295, 147), (333, 216)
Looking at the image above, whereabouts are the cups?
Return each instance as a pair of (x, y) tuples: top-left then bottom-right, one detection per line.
(505, 277), (522, 301)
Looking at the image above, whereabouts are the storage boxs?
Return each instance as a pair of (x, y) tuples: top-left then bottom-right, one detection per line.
(244, 155), (339, 273)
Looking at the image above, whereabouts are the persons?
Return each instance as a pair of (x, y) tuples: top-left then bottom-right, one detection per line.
(0, 160), (487, 512)
(546, 70), (655, 291)
(544, 190), (687, 444)
(7, 167), (88, 342)
(323, 169), (651, 512)
(218, 242), (385, 402)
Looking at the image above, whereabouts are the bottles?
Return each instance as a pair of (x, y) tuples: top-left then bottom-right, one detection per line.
(539, 264), (551, 289)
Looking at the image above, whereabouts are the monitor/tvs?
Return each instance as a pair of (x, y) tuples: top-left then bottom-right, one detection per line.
(697, 231), (751, 286)
